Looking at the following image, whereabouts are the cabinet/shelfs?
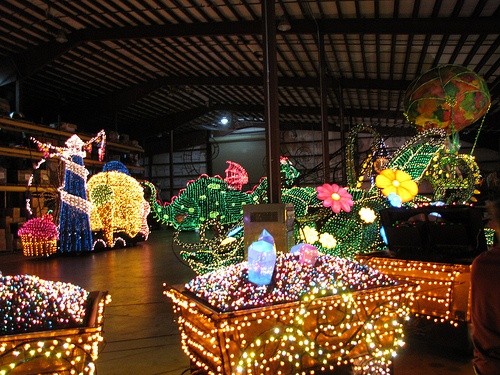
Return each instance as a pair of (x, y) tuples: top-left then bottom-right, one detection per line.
(0, 97), (214, 251)
